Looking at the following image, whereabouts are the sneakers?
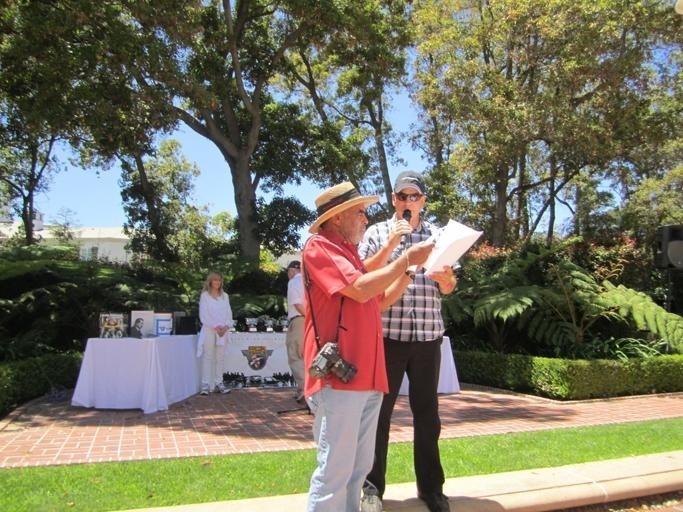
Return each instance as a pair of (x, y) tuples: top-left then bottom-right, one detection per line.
(200, 386), (209, 395)
(215, 383), (232, 394)
(360, 490), (383, 512)
(417, 492), (450, 512)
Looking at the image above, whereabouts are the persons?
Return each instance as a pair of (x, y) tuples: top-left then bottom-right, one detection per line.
(196, 273), (234, 396)
(287, 261), (304, 401)
(358, 171), (461, 512)
(303, 182), (435, 512)
(130, 319), (144, 338)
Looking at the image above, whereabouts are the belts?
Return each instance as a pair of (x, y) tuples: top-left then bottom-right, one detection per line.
(291, 315), (303, 320)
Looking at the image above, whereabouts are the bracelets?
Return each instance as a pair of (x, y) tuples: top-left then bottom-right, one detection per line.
(404, 251), (410, 269)
(405, 270), (415, 280)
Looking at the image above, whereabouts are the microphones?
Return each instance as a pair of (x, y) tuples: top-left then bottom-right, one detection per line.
(399, 209), (411, 244)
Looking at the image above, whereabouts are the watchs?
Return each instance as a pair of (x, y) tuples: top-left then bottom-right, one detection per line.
(438, 280), (453, 290)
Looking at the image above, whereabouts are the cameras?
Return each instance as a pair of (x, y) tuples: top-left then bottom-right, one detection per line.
(309, 342), (357, 384)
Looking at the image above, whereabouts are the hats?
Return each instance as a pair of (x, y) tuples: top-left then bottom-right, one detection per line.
(393, 170), (425, 194)
(308, 181), (380, 233)
(286, 260), (300, 272)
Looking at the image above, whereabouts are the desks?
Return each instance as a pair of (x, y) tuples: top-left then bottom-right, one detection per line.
(70, 334), (460, 415)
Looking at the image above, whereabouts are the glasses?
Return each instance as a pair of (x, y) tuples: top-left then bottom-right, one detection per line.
(396, 192), (420, 202)
